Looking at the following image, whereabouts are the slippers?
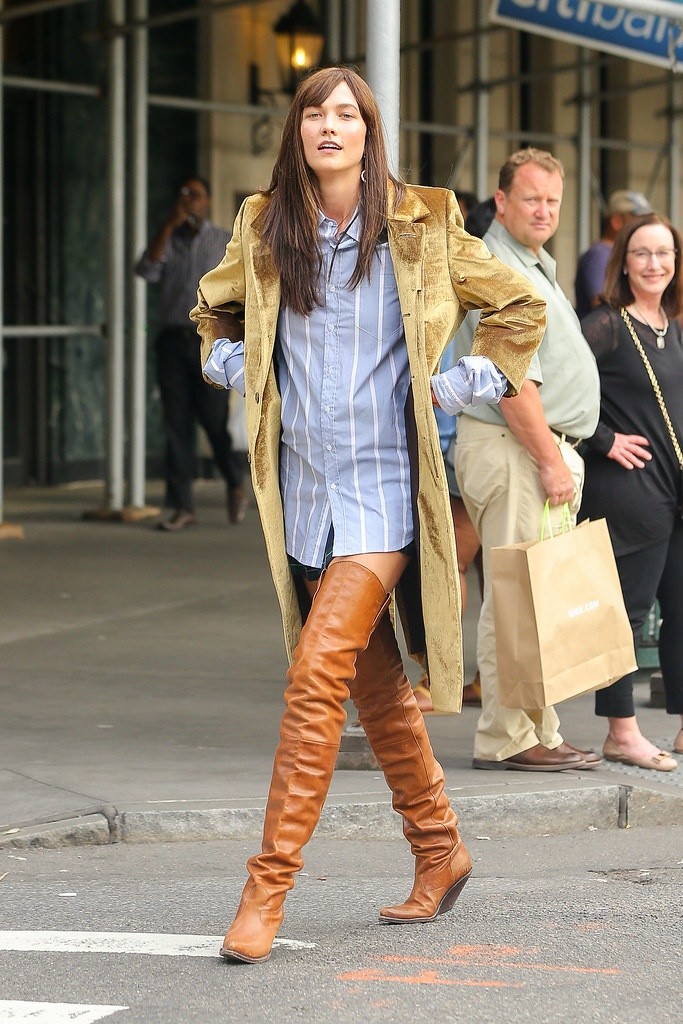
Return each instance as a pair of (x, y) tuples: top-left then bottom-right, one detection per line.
(462, 682), (483, 706)
(411, 673), (459, 715)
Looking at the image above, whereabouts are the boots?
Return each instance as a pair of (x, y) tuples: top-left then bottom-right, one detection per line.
(219, 561), (394, 964)
(302, 577), (475, 924)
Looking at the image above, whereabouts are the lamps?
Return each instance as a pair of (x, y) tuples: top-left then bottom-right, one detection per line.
(249, 0), (328, 106)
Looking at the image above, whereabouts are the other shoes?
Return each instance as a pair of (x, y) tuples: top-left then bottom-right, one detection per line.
(225, 485), (250, 528)
(157, 509), (196, 531)
(602, 735), (678, 771)
(674, 729), (683, 753)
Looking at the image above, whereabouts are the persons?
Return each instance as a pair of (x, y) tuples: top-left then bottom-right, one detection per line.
(454, 147), (605, 772)
(395, 191), (499, 716)
(189, 67), (549, 963)
(576, 212), (683, 771)
(576, 190), (652, 321)
(136, 178), (248, 531)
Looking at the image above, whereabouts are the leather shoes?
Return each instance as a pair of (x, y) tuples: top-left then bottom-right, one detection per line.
(472, 744), (586, 771)
(553, 742), (604, 769)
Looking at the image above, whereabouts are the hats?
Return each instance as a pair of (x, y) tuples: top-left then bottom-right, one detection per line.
(607, 189), (661, 218)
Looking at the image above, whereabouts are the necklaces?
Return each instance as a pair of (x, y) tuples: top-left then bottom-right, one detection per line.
(633, 303), (669, 349)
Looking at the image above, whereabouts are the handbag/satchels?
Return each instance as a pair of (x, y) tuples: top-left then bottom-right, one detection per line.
(490, 496), (641, 711)
